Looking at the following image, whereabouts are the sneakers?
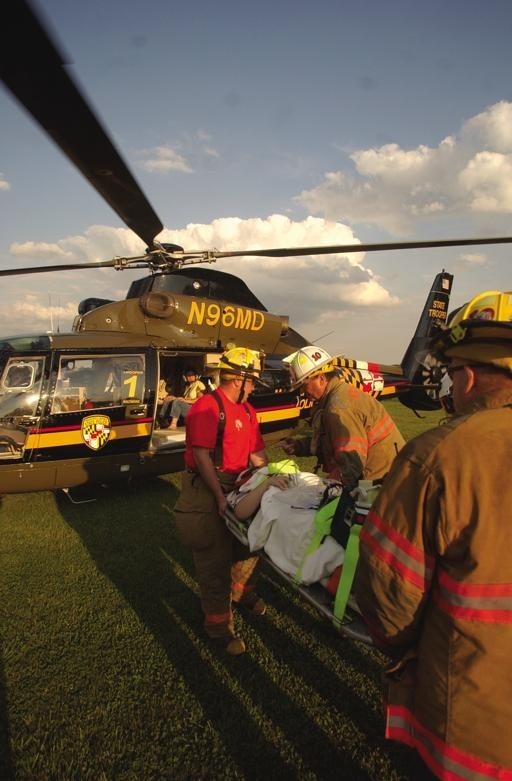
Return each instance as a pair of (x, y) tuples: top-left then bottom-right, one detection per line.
(233, 590), (267, 616)
(217, 631), (245, 656)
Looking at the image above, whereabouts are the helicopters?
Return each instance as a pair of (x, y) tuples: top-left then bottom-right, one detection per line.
(0, 0), (511, 503)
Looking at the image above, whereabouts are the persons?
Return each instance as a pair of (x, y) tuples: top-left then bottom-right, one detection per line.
(357, 287), (510, 781)
(281, 344), (409, 487)
(153, 364), (206, 429)
(174, 345), (271, 655)
(224, 463), (292, 522)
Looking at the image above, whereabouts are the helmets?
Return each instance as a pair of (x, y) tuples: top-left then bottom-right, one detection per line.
(282, 345), (344, 392)
(429, 291), (512, 366)
(206, 346), (270, 389)
